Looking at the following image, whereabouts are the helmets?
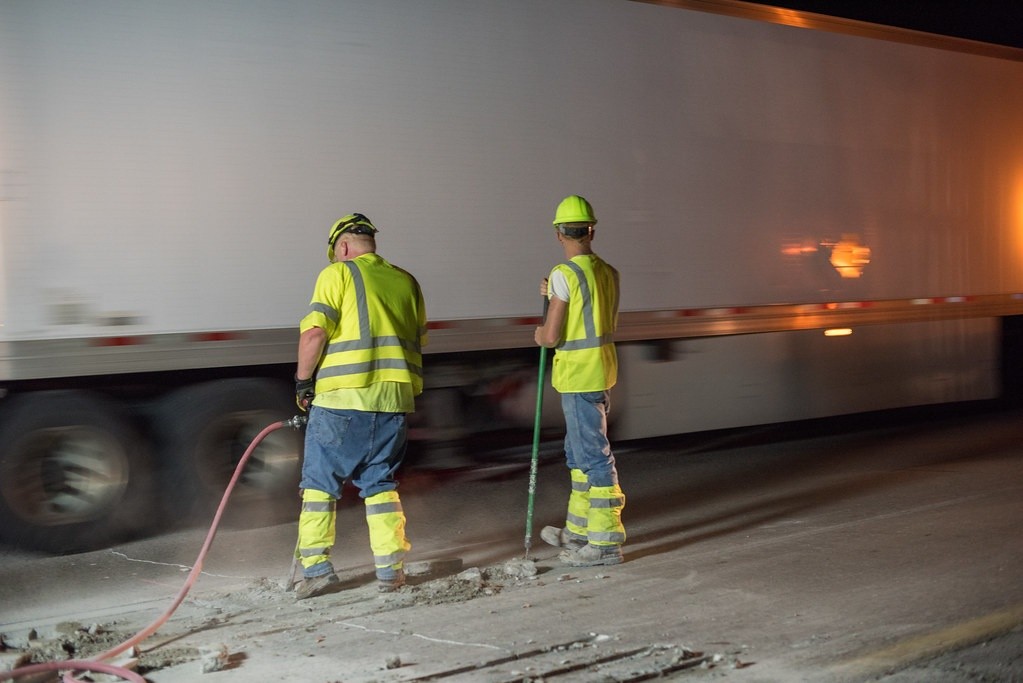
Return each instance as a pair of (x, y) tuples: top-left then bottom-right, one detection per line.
(553, 195), (598, 235)
(327, 213), (379, 263)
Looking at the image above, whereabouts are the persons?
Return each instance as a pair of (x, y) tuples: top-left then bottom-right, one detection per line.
(534, 193), (628, 568)
(293, 212), (428, 600)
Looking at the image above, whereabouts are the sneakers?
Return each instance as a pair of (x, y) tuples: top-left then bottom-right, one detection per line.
(377, 569), (406, 593)
(559, 542), (624, 567)
(296, 571), (339, 600)
(540, 526), (588, 552)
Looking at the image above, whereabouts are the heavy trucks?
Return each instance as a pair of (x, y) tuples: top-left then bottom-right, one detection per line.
(0, 1), (1023, 558)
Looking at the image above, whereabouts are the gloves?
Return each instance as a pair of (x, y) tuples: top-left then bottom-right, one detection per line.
(295, 373), (315, 411)
